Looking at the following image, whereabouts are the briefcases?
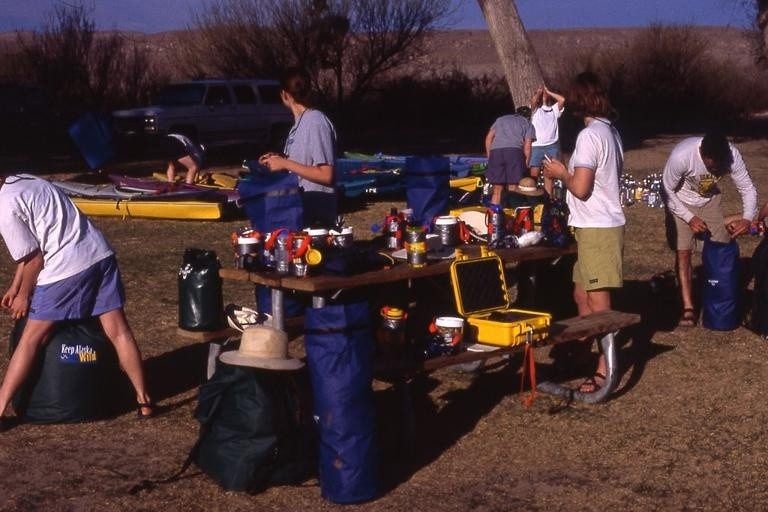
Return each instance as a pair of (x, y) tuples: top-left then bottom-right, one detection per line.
(448, 244), (554, 348)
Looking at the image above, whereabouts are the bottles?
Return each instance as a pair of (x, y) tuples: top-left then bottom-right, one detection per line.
(488, 205), (505, 247)
(290, 235), (322, 277)
(385, 208), (403, 251)
(275, 231), (289, 275)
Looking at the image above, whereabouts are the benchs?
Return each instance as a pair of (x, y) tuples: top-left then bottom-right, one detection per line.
(374, 309), (642, 447)
(178, 290), (404, 395)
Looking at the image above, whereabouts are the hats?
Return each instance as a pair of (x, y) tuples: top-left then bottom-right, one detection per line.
(218, 324), (306, 371)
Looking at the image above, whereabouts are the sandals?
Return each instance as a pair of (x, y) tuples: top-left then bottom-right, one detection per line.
(137, 402), (153, 419)
(680, 307), (695, 328)
(578, 372), (605, 393)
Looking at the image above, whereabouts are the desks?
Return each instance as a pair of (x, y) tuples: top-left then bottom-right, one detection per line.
(218, 234), (578, 330)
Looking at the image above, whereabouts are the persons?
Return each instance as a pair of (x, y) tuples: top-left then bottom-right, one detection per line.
(658, 132), (759, 328)
(0, 173), (156, 428)
(530, 86), (566, 198)
(543, 73), (627, 392)
(484, 106), (537, 204)
(162, 137), (204, 183)
(258, 73), (340, 233)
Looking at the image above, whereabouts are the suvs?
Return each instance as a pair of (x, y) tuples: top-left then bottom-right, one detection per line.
(113, 76), (298, 152)
